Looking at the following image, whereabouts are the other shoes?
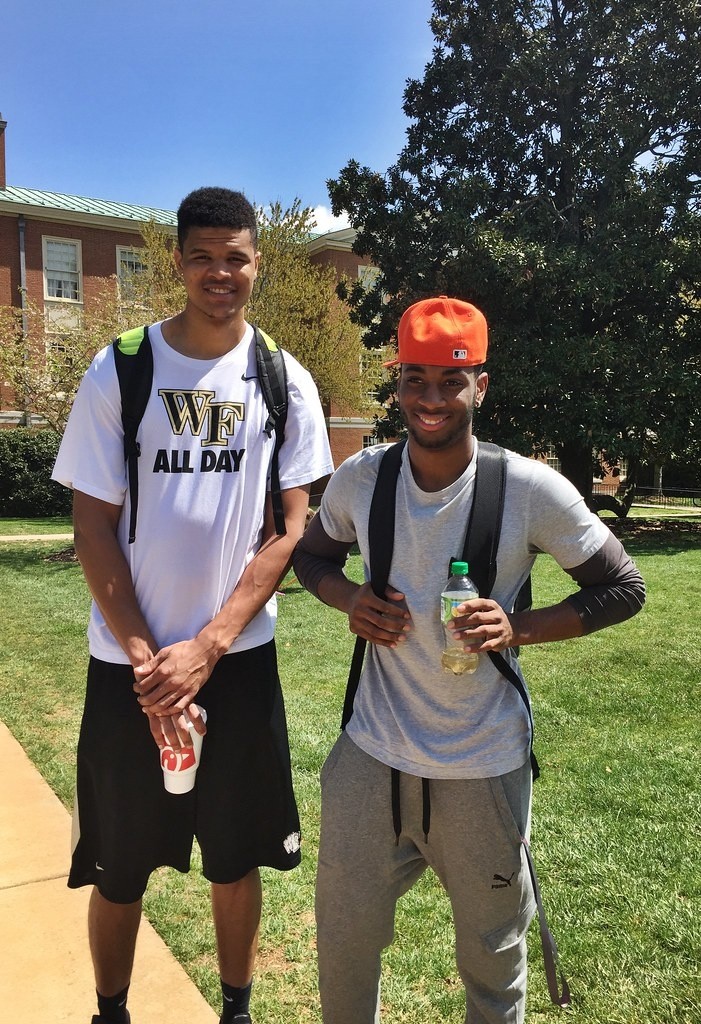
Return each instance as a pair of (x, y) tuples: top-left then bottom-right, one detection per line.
(219, 1012), (252, 1024)
(92, 1009), (130, 1024)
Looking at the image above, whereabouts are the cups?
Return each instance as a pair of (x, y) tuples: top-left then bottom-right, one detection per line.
(160, 703), (207, 794)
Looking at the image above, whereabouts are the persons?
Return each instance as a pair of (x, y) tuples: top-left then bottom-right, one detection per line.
(49, 188), (335, 1024)
(288, 293), (644, 1024)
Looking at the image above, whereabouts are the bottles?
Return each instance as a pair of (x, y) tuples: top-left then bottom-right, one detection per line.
(440, 561), (480, 678)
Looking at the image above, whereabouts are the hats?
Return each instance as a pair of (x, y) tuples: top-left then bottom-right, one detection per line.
(382, 296), (488, 368)
